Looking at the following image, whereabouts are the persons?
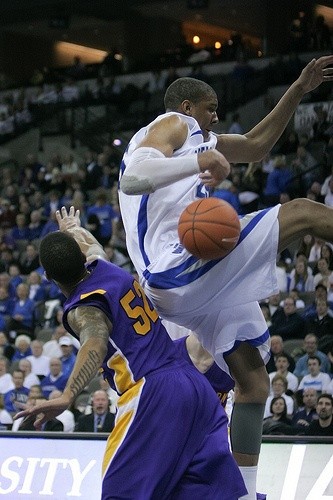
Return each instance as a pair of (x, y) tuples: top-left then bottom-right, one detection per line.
(118, 56), (333, 500)
(13, 206), (250, 500)
(0, 15), (332, 437)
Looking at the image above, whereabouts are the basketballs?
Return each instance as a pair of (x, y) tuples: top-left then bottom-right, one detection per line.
(177, 196), (242, 261)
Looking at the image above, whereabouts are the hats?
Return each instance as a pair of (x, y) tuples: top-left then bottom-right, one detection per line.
(59, 336), (72, 346)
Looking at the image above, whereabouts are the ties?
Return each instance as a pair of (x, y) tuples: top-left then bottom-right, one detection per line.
(96, 417), (101, 432)
